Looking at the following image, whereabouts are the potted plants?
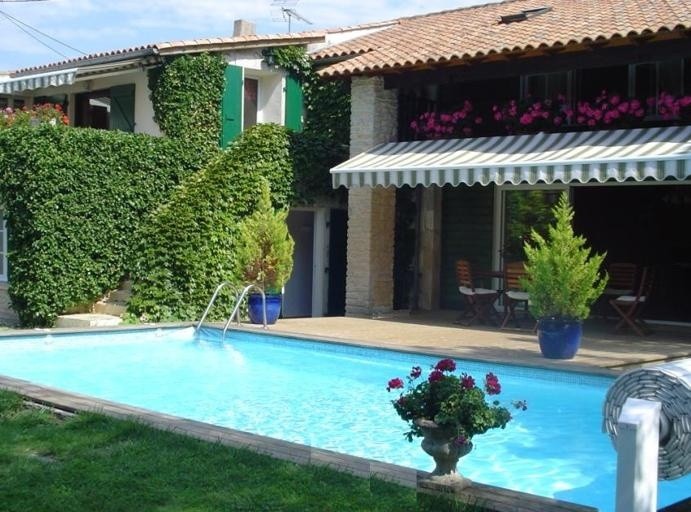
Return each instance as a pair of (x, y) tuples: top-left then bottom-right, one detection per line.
(514, 184), (612, 361)
(230, 174), (298, 326)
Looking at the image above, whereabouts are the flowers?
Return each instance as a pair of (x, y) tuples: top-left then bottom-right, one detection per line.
(387, 353), (530, 456)
(407, 87), (691, 140)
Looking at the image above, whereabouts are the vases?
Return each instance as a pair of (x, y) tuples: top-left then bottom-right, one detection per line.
(414, 419), (474, 491)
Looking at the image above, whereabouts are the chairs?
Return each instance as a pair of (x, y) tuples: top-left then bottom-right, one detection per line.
(608, 264), (658, 337)
(454, 258), (502, 327)
(501, 261), (638, 330)
